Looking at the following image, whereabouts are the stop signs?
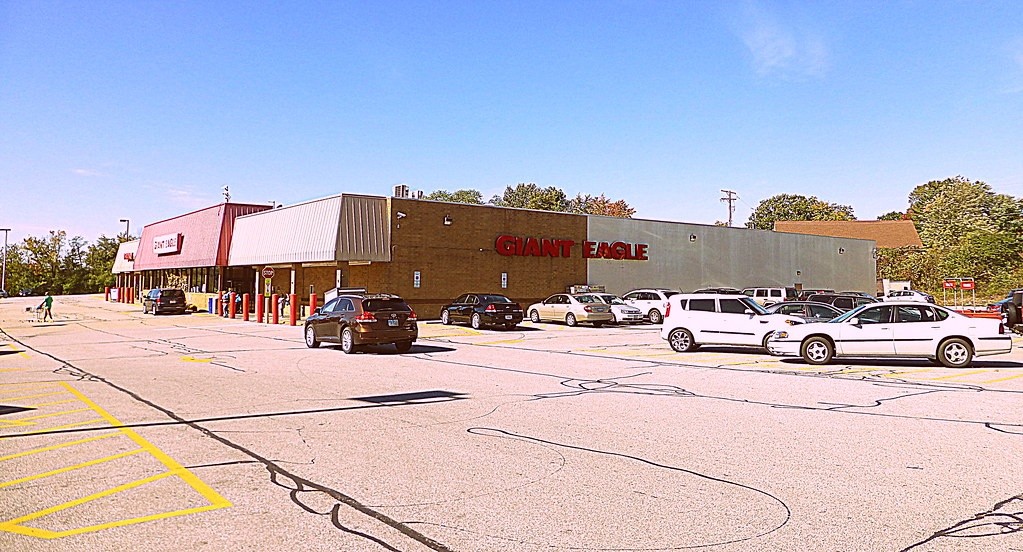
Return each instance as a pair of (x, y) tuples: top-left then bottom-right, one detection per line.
(262, 266), (275, 279)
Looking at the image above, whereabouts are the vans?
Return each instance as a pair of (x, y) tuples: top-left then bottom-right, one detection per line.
(143, 289), (187, 316)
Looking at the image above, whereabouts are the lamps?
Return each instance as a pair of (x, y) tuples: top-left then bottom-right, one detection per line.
(444, 214), (453, 225)
(690, 233), (696, 242)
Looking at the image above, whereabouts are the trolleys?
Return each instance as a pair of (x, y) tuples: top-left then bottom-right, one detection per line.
(25, 305), (45, 323)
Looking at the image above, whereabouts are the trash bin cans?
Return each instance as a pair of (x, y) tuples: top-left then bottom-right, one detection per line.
(209, 298), (218, 315)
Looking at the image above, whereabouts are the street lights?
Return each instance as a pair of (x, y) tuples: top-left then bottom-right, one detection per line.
(0, 228), (11, 290)
(120, 219), (129, 242)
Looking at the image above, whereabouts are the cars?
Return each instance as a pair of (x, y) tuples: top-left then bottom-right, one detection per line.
(764, 301), (879, 323)
(876, 290), (936, 304)
(439, 292), (525, 330)
(768, 301), (1012, 368)
(584, 293), (644, 324)
(840, 291), (879, 302)
(797, 289), (836, 298)
(527, 293), (612, 327)
(660, 293), (806, 357)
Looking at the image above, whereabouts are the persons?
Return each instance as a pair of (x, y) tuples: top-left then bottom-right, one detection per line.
(36, 290), (53, 324)
(220, 287), (241, 320)
(278, 293), (290, 318)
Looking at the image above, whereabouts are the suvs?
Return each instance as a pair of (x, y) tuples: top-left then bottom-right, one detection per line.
(621, 288), (680, 324)
(741, 286), (802, 315)
(304, 293), (419, 354)
(692, 287), (742, 295)
(1001, 291), (1023, 337)
(805, 293), (920, 323)
